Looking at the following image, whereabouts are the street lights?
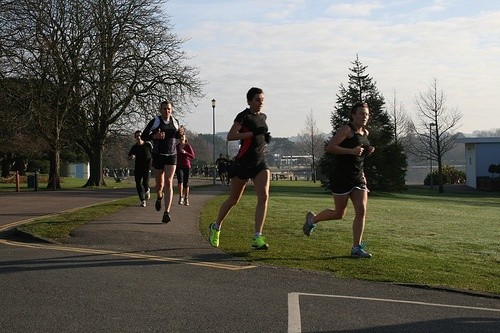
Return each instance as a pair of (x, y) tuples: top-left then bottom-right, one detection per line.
(208, 97), (217, 184)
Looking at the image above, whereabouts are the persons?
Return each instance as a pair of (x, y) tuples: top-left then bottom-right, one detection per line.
(208, 87), (272, 250)
(140, 100), (186, 224)
(175, 132), (195, 206)
(214, 153), (232, 188)
(302, 101), (375, 258)
(127, 129), (153, 208)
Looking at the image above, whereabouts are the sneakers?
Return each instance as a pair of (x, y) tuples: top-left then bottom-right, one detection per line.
(303, 211), (316, 235)
(351, 245), (372, 257)
(209, 221), (221, 247)
(252, 234), (269, 249)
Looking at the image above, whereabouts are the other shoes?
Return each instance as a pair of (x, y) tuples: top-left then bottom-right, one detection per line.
(141, 200), (147, 207)
(145, 187), (151, 199)
(184, 197), (190, 205)
(178, 196), (184, 204)
(155, 193), (164, 210)
(162, 211), (172, 223)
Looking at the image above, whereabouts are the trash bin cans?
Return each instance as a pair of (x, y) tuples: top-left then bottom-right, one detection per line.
(27, 175), (35, 188)
